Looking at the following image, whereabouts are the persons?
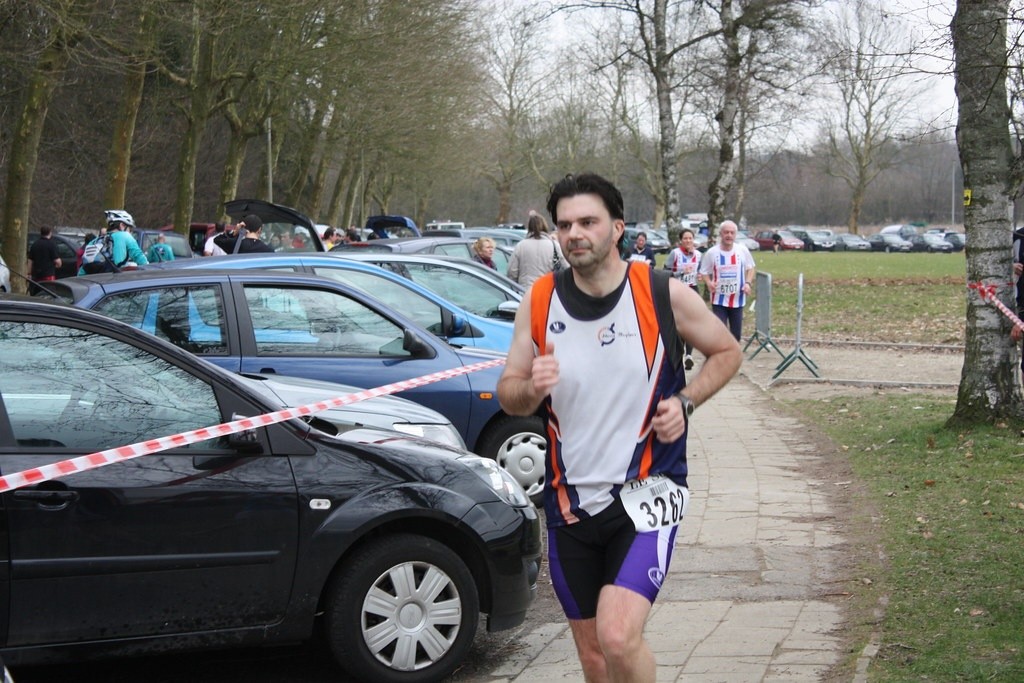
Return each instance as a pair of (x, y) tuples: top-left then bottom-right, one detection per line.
(27, 225), (62, 290)
(662, 229), (703, 370)
(507, 213), (570, 294)
(699, 220), (754, 348)
(147, 233), (175, 265)
(263, 226), (412, 255)
(495, 172), (742, 683)
(471, 237), (497, 272)
(77, 227), (108, 275)
(213, 216), (274, 255)
(1012, 226), (1024, 436)
(78, 210), (150, 278)
(771, 230), (781, 255)
(204, 221), (229, 257)
(622, 232), (656, 270)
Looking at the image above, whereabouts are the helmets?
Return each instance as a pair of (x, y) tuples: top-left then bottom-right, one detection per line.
(105, 209), (136, 228)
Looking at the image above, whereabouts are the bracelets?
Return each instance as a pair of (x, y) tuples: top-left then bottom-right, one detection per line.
(746, 282), (751, 286)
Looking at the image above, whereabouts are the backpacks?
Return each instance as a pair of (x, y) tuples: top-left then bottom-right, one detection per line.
(82, 233), (129, 273)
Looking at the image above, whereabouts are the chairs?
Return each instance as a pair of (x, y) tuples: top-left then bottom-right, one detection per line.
(159, 311), (201, 351)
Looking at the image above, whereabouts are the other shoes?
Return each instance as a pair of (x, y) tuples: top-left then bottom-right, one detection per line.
(685, 357), (693, 371)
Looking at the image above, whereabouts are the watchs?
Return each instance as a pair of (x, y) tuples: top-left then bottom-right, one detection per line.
(674, 392), (694, 418)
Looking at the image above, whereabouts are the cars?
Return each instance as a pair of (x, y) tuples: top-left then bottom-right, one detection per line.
(927, 227), (966, 252)
(835, 234), (872, 251)
(26, 223), (234, 279)
(0, 293), (543, 682)
(881, 224), (918, 240)
(868, 234), (913, 253)
(0, 257), (10, 293)
(224, 198), (528, 276)
(35, 272), (550, 509)
(625, 228), (672, 256)
(903, 234), (955, 253)
(163, 224), (236, 255)
(127, 251), (515, 354)
(338, 253), (521, 322)
(735, 232), (759, 252)
(750, 230), (805, 252)
(418, 254), (526, 296)
(791, 229), (836, 252)
